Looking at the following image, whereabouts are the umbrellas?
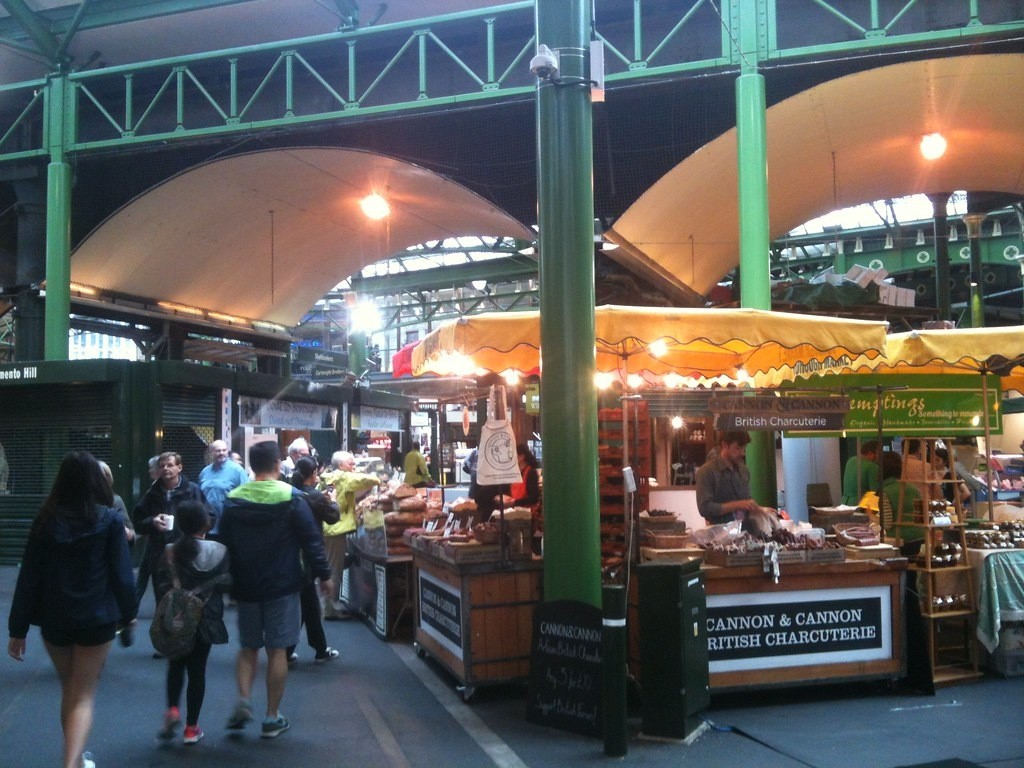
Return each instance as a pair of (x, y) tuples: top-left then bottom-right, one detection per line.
(391, 302), (890, 556)
(754, 324), (1024, 532)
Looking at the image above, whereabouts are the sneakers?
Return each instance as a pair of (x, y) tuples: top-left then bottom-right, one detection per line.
(224, 705), (253, 730)
(262, 714), (290, 737)
(183, 725), (204, 743)
(288, 652), (298, 664)
(158, 707), (181, 741)
(315, 647), (339, 663)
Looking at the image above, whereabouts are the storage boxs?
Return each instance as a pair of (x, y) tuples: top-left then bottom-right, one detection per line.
(842, 264), (877, 289)
(906, 289), (916, 307)
(871, 267), (889, 286)
(897, 287), (907, 307)
(888, 285), (896, 306)
(877, 282), (889, 306)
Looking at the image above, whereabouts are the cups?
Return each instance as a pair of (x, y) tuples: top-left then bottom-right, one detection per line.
(163, 515), (174, 530)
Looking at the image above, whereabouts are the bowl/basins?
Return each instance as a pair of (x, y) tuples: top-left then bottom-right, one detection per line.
(472, 523), (499, 543)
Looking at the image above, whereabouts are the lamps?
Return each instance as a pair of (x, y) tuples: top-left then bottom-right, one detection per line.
(342, 291), (359, 309)
(971, 272), (978, 286)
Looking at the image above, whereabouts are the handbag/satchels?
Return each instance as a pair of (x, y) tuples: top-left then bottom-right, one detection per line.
(476, 386), (523, 485)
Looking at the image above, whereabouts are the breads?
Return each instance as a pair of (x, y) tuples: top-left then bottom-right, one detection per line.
(354, 481), (476, 556)
(597, 406), (650, 559)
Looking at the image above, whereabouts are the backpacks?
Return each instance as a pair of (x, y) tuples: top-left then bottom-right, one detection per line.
(148, 543), (227, 663)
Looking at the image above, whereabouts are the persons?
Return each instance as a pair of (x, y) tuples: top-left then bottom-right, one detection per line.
(882, 452), (926, 557)
(287, 457), (339, 665)
(97, 461), (135, 549)
(374, 344), (380, 357)
(462, 449), (493, 512)
(153, 499), (229, 745)
(493, 444), (540, 555)
(279, 438), (311, 486)
(316, 450), (382, 621)
(7, 453), (138, 768)
(936, 448), (971, 508)
(229, 451), (243, 466)
(902, 439), (939, 504)
(200, 440), (250, 538)
(841, 440), (880, 504)
(221, 441), (334, 739)
(403, 441), (432, 487)
(132, 451), (215, 606)
(695, 430), (776, 525)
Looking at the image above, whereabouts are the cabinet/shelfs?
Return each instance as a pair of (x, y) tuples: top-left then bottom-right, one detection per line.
(892, 435), (984, 696)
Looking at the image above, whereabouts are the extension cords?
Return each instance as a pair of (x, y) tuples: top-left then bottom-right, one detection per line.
(622, 467), (637, 493)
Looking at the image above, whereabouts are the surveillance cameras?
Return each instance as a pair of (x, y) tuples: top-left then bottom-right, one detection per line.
(530, 52), (558, 80)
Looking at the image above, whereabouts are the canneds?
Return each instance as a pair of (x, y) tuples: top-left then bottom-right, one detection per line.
(913, 498), (1024, 613)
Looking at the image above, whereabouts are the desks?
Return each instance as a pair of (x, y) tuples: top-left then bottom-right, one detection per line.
(918, 547), (1024, 678)
(348, 536), (415, 640)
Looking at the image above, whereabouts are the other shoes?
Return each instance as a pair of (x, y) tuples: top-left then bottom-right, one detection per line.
(83, 751), (95, 768)
(337, 607), (352, 618)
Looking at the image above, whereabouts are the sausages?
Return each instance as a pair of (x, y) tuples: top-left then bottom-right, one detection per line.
(697, 527), (839, 554)
